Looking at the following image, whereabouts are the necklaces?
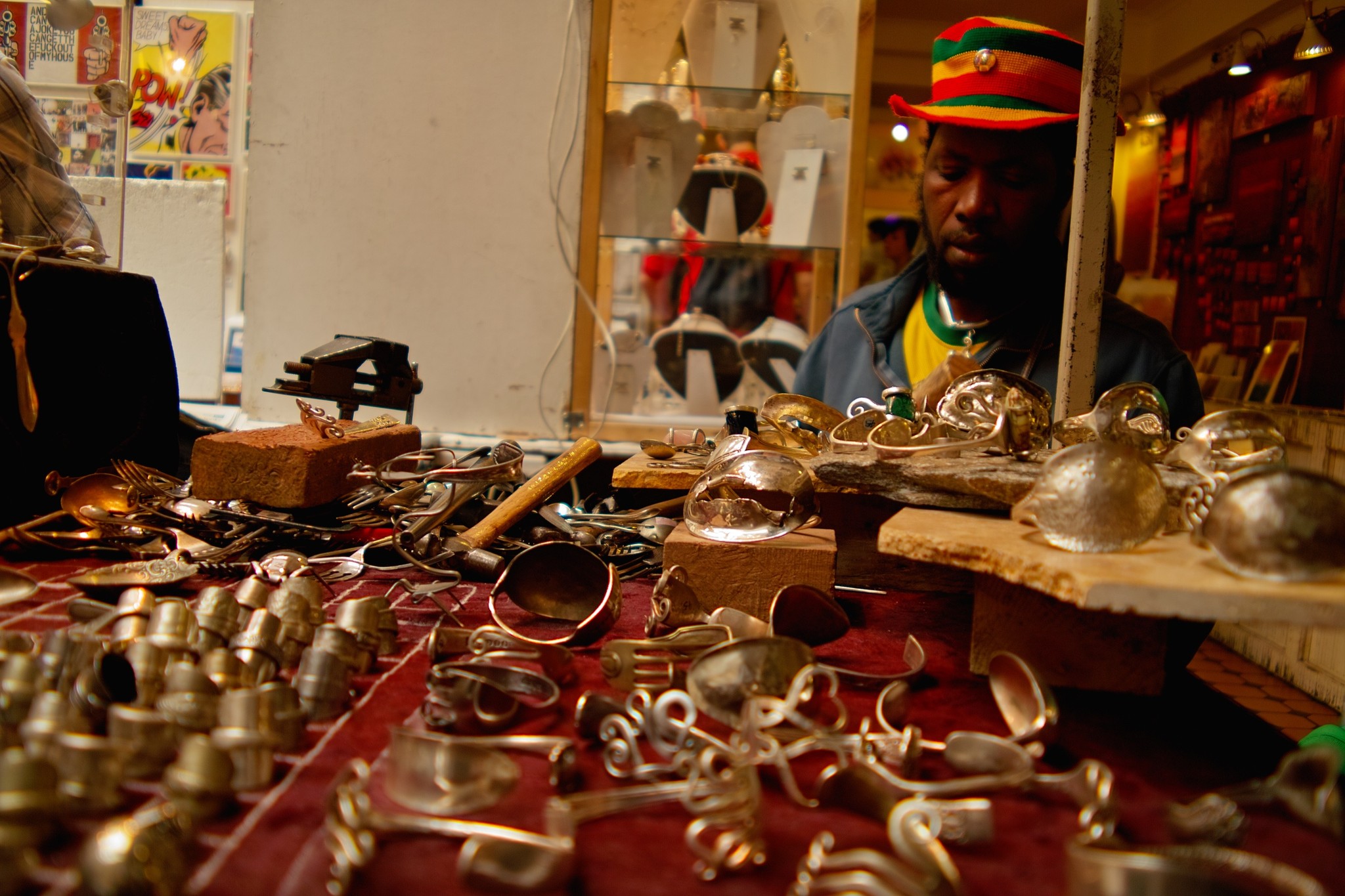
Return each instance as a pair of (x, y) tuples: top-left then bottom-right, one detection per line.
(675, 156), (775, 361)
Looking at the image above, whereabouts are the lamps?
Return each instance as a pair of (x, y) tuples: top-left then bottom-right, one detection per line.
(1137, 78), (1168, 127)
(1227, 27), (1267, 76)
(1293, 0), (1333, 60)
(1117, 91), (1141, 130)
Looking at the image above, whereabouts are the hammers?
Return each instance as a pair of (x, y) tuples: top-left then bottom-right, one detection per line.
(412, 437), (603, 577)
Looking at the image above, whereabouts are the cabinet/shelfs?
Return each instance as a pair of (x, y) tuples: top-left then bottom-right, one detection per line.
(571, 0), (873, 442)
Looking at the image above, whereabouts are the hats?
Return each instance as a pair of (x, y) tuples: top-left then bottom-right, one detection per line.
(890, 15), (1127, 136)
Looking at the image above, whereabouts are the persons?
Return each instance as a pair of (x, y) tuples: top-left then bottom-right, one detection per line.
(790, 15), (1204, 440)
(0, 51), (107, 257)
